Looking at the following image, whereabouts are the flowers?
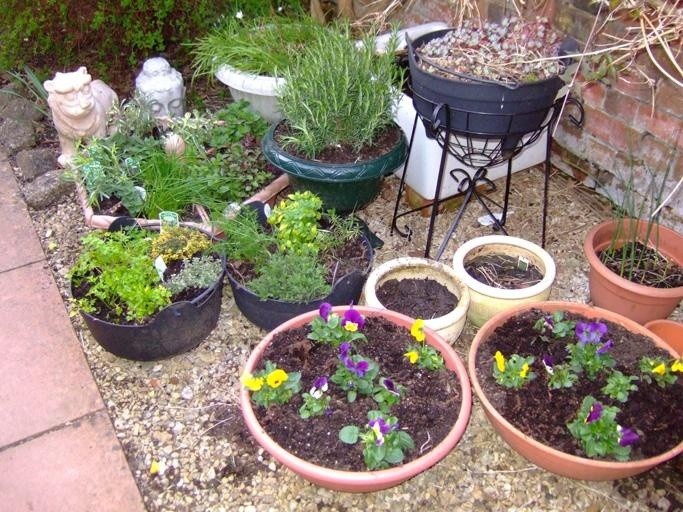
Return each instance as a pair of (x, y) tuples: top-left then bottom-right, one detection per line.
(490, 311), (682, 464)
(243, 302), (451, 470)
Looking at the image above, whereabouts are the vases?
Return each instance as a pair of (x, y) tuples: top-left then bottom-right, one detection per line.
(363, 256), (469, 347)
(238, 304), (472, 493)
(452, 234), (557, 329)
(466, 302), (682, 480)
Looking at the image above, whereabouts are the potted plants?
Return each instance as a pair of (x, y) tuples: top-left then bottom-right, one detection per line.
(260, 17), (406, 215)
(401, 12), (568, 140)
(583, 123), (683, 325)
(189, 23), (331, 122)
(225, 203), (373, 331)
(70, 216), (224, 362)
(70, 117), (290, 241)
